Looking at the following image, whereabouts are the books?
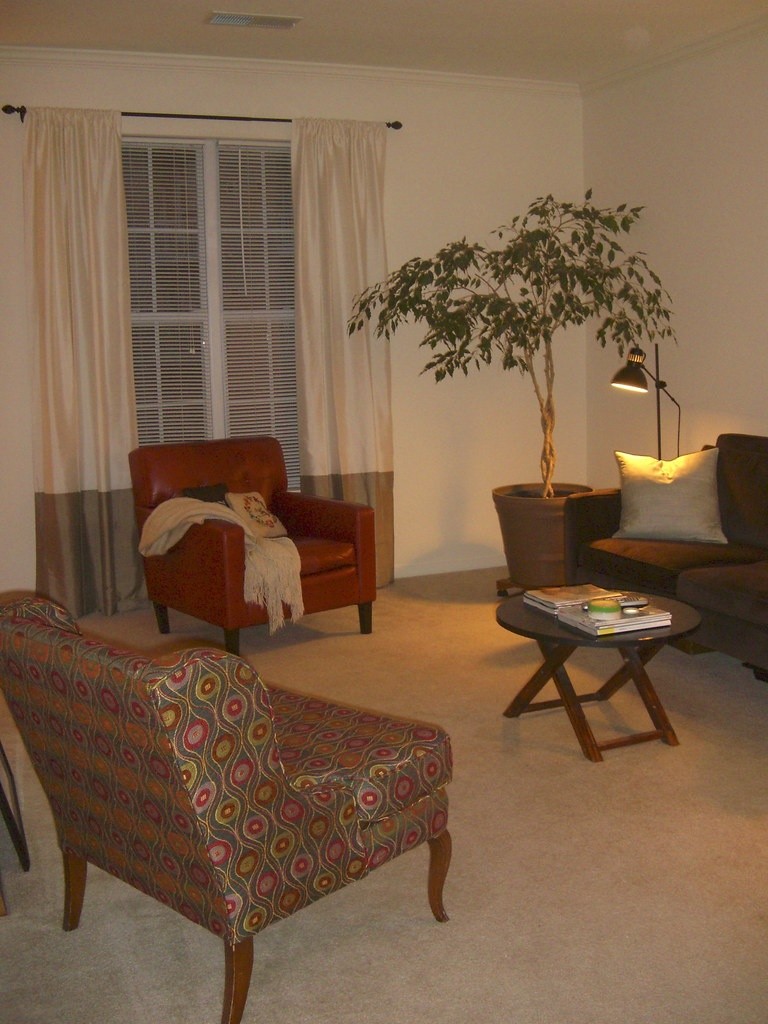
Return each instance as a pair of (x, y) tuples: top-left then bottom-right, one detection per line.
(523, 583), (673, 636)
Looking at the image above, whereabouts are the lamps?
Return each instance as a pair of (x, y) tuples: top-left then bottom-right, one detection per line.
(611, 344), (680, 456)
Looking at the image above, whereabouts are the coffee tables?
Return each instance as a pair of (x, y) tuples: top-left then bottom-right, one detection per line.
(496, 591), (702, 762)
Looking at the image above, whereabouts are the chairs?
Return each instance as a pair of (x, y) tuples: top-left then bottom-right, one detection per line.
(0, 597), (453, 1024)
(128, 437), (376, 656)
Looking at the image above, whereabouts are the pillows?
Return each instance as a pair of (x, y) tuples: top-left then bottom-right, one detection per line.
(225, 491), (287, 537)
(184, 483), (229, 510)
(612, 447), (728, 544)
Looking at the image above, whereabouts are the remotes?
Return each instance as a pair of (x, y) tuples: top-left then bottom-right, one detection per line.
(581, 596), (649, 612)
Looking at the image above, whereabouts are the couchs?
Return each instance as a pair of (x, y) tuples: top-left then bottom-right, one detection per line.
(563, 434), (768, 671)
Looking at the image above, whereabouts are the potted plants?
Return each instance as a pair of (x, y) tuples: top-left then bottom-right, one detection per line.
(348, 186), (677, 586)
